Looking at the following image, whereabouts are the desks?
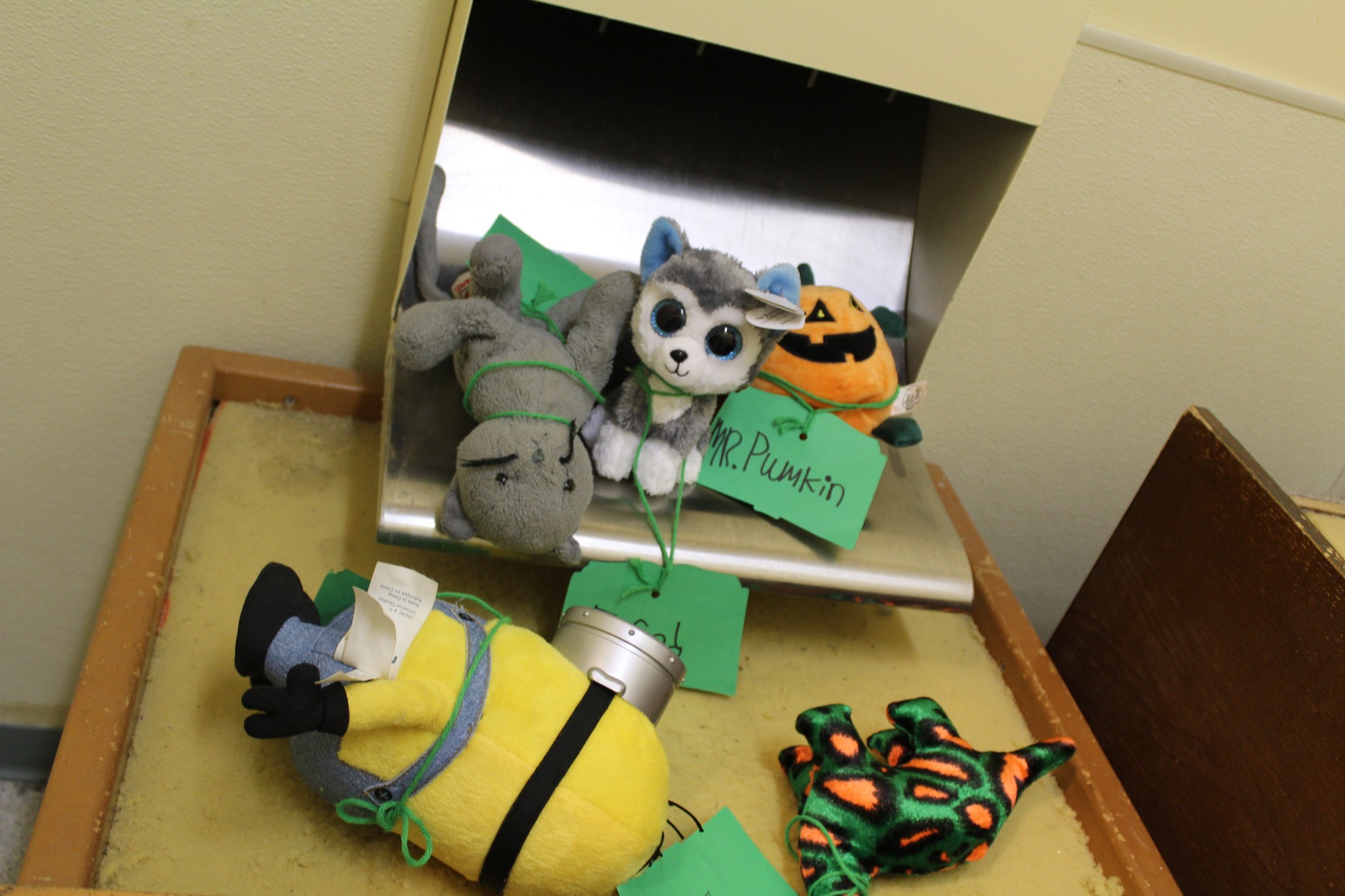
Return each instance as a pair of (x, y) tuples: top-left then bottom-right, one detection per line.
(15, 346), (1183, 896)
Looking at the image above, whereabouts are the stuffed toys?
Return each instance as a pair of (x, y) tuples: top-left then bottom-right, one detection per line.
(234, 555), (684, 896)
(752, 263), (924, 449)
(580, 215), (804, 496)
(389, 163), (640, 568)
